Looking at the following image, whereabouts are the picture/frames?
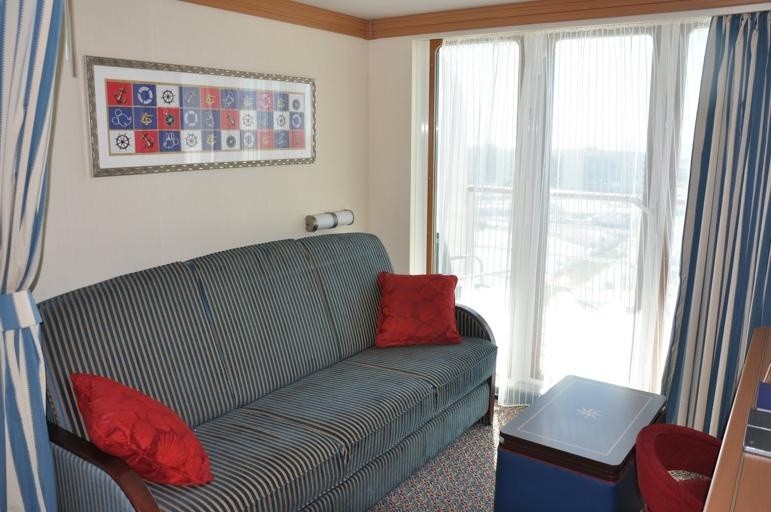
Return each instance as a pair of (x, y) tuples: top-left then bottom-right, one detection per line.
(82, 55), (317, 179)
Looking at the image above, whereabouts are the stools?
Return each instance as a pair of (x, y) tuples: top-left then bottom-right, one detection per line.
(635, 422), (724, 512)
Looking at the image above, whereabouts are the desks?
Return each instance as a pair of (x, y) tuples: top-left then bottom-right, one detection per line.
(494, 374), (668, 512)
(702, 326), (771, 510)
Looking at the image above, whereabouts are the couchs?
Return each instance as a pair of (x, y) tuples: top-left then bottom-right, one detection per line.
(31, 232), (498, 511)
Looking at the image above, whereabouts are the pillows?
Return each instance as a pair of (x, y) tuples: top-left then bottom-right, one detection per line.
(375, 270), (464, 348)
(68, 372), (216, 488)
